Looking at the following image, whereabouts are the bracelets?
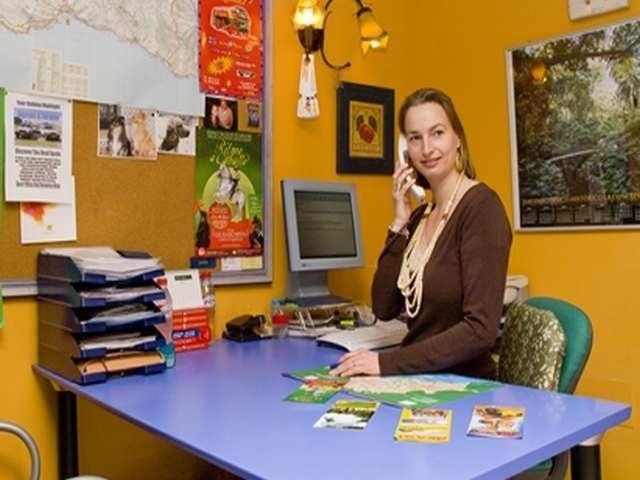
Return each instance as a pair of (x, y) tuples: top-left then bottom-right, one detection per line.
(386, 224), (410, 239)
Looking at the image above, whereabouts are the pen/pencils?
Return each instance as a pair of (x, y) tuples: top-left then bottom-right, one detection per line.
(323, 361), (341, 373)
(334, 320), (360, 326)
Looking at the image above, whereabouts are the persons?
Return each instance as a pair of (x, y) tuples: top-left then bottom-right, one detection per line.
(218, 100), (233, 131)
(206, 104), (219, 130)
(328, 89), (512, 379)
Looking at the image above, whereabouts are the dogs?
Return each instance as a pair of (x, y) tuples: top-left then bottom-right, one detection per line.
(129, 110), (155, 156)
(104, 115), (131, 157)
(156, 113), (190, 152)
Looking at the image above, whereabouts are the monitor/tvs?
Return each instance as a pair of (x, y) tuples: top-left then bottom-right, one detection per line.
(273, 179), (364, 310)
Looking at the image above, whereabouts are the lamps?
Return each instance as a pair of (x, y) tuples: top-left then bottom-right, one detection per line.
(291, 0), (391, 70)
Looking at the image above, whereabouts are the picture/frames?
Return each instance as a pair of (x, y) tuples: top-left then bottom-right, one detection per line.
(501, 17), (640, 235)
(335, 79), (395, 175)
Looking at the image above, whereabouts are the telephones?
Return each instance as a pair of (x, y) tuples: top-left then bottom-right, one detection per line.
(398, 134), (425, 202)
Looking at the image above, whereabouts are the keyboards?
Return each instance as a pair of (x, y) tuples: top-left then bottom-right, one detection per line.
(316, 319), (408, 351)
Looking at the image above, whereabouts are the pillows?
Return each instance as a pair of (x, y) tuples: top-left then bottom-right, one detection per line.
(497, 301), (566, 393)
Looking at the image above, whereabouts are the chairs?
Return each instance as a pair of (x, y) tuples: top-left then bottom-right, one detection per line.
(1, 422), (110, 480)
(493, 296), (594, 479)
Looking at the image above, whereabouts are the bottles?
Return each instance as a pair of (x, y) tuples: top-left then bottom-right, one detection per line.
(153, 278), (177, 368)
(197, 270), (218, 346)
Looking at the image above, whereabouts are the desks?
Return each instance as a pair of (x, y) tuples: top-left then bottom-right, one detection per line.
(31, 307), (631, 479)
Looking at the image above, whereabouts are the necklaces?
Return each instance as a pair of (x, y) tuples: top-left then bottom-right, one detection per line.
(396, 171), (463, 319)
